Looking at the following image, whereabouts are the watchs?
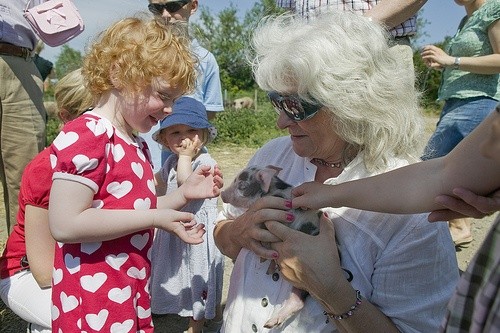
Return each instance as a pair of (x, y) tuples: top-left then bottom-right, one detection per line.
(454, 56), (460, 70)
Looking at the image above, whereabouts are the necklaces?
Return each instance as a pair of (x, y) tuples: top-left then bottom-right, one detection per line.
(312, 158), (344, 168)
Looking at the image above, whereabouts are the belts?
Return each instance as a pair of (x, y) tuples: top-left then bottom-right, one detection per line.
(0, 42), (33, 60)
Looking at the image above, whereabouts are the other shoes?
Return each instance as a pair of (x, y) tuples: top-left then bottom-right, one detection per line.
(448, 226), (474, 247)
(26, 322), (39, 333)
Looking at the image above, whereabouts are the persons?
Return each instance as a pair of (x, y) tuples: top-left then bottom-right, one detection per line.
(0, 0), (500, 333)
(47, 12), (224, 333)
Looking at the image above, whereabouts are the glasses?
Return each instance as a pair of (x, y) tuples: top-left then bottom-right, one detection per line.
(148, 0), (191, 15)
(267, 91), (324, 121)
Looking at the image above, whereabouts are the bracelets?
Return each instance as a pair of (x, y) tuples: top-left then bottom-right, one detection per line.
(322, 290), (362, 323)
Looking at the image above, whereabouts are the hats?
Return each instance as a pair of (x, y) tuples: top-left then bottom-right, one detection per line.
(152, 97), (218, 148)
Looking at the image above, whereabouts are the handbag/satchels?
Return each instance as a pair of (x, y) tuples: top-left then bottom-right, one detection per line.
(22, 0), (85, 47)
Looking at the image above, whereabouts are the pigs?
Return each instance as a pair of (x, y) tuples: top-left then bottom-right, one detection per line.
(233, 97), (253, 109)
(220, 164), (327, 331)
